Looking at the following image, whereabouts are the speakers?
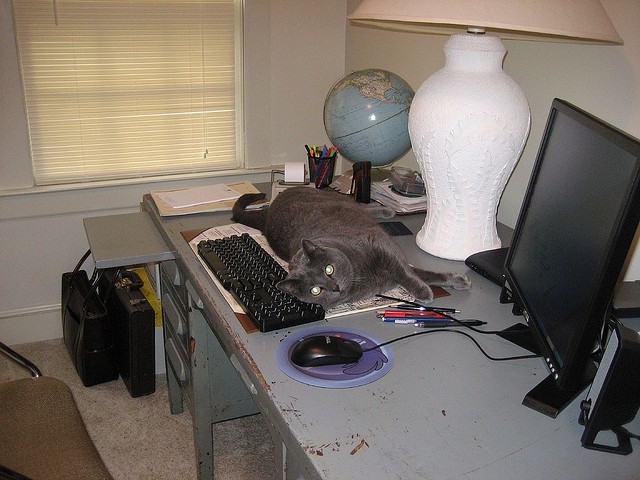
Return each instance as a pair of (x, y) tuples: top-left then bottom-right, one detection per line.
(578, 324), (640, 456)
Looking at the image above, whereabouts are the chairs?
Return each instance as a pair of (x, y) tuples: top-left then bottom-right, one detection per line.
(0, 341), (112, 480)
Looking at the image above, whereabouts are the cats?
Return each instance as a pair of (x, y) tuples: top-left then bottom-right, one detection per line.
(232, 186), (472, 309)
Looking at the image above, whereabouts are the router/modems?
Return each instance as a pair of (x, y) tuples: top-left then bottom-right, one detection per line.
(464, 246), (510, 287)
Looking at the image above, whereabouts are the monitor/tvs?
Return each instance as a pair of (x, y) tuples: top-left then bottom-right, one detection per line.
(497, 97), (640, 387)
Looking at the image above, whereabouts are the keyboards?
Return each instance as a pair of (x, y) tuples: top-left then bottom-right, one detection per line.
(196, 233), (325, 333)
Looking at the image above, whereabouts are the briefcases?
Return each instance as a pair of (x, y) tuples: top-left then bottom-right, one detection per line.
(104, 267), (155, 397)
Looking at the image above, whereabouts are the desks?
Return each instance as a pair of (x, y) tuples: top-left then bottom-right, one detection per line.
(81, 172), (640, 479)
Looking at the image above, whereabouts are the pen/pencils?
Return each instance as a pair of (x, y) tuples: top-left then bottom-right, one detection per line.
(376, 313), (446, 318)
(389, 304), (461, 313)
(376, 310), (447, 314)
(305, 144), (338, 189)
(382, 317), (453, 323)
(415, 319), (487, 328)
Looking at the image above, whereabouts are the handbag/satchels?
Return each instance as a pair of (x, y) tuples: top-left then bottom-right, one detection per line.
(62, 249), (119, 387)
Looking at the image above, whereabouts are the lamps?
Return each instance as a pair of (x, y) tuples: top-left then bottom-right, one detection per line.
(348, 1), (625, 263)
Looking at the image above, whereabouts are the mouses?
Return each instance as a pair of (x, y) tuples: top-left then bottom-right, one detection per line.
(291, 336), (363, 367)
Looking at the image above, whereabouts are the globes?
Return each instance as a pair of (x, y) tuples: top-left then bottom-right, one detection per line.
(322, 68), (416, 195)
